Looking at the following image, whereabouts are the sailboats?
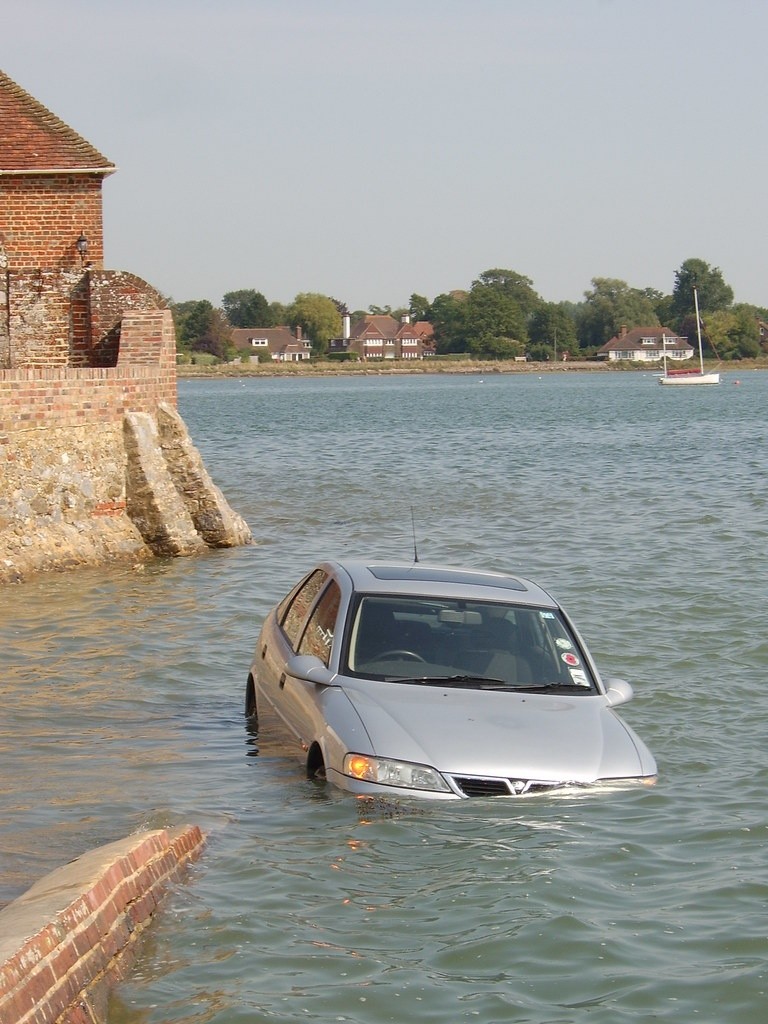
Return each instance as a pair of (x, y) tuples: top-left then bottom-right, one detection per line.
(659, 285), (720, 385)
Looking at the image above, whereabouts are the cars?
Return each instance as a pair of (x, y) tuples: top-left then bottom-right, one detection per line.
(242, 560), (658, 807)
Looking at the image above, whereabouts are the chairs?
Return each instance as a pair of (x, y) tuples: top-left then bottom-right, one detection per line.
(360, 605), (403, 660)
(448, 618), (534, 682)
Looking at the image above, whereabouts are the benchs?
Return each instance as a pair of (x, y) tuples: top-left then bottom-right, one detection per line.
(514, 356), (526, 362)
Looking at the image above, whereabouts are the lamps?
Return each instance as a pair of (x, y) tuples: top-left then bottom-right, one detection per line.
(33, 270), (45, 296)
(77, 230), (88, 261)
